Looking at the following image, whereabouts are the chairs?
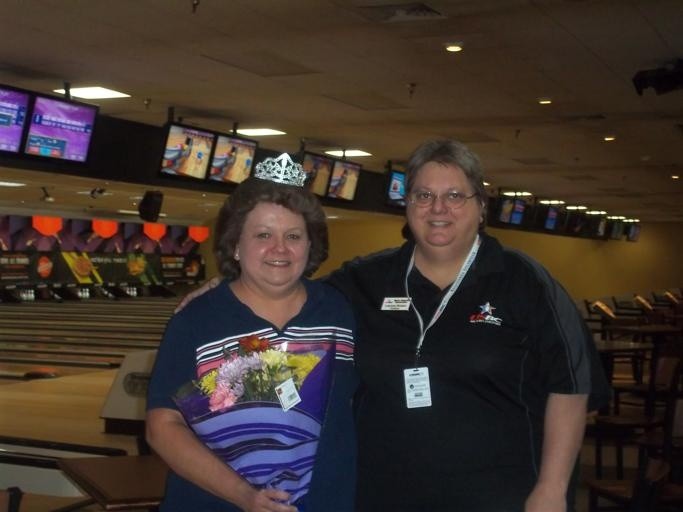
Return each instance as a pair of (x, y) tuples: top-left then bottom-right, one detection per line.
(575, 289), (683, 512)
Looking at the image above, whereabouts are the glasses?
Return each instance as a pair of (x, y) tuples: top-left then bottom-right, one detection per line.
(405, 190), (478, 209)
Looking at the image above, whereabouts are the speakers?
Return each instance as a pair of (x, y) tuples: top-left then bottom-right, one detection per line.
(139, 190), (164, 223)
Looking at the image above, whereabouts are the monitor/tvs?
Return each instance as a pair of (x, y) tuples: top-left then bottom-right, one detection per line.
(489, 195), (641, 243)
(0, 83), (100, 168)
(156, 121), (407, 211)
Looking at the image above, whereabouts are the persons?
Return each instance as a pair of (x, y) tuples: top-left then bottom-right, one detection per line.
(169, 138), (615, 511)
(143, 148), (361, 512)
